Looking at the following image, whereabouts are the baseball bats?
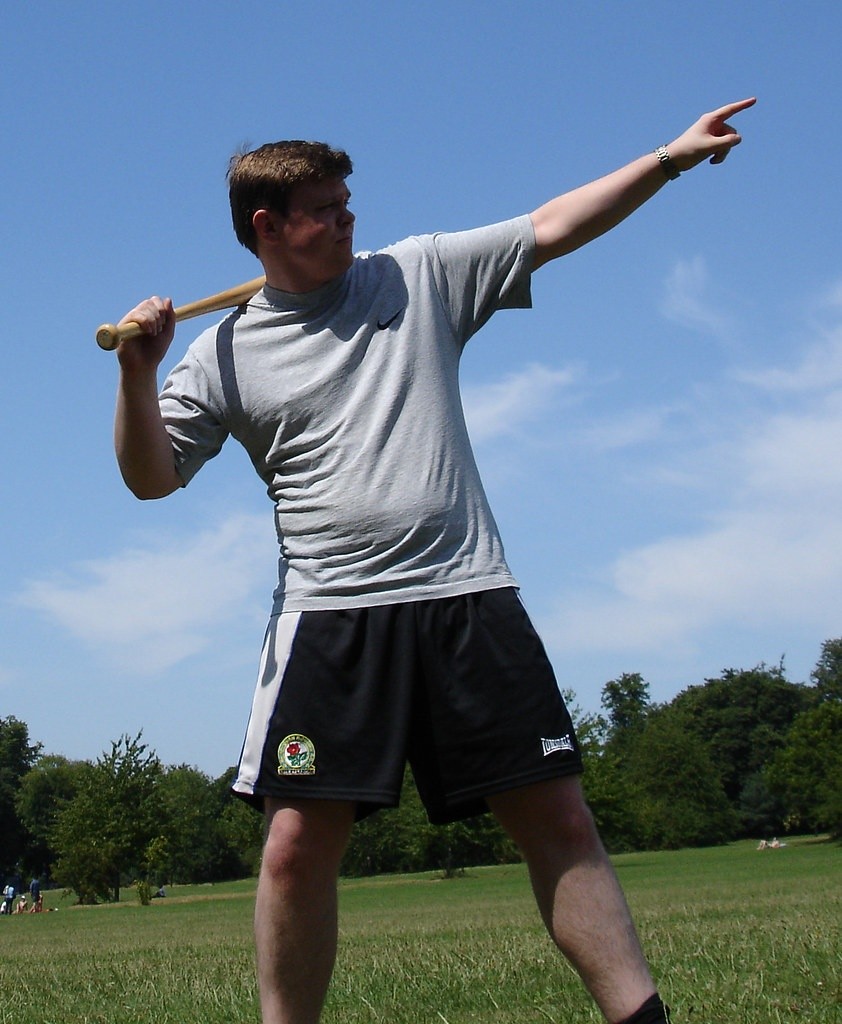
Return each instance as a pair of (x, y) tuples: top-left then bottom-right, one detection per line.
(96, 274), (267, 351)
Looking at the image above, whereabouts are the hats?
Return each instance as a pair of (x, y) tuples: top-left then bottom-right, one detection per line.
(21, 896), (26, 899)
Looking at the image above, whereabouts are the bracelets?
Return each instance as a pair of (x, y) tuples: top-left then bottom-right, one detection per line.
(655, 144), (680, 180)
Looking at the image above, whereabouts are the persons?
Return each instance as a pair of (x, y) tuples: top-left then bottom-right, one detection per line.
(13, 896), (28, 914)
(757, 837), (779, 850)
(0, 899), (6, 914)
(116, 97), (759, 1024)
(29, 895), (43, 913)
(5, 885), (15, 914)
(150, 883), (165, 898)
(29, 875), (40, 912)
